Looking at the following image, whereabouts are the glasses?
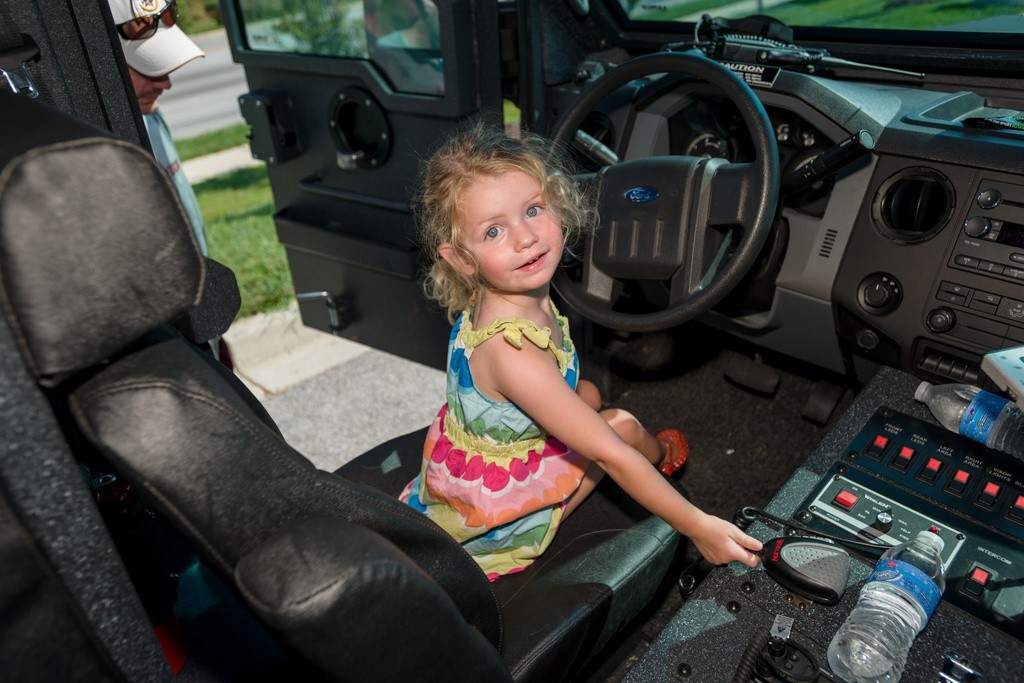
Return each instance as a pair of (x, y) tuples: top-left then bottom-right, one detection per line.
(115, 1), (179, 41)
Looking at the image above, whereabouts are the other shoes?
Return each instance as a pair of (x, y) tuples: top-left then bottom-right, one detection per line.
(657, 429), (688, 475)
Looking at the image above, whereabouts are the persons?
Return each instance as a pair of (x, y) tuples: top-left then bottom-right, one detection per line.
(397, 127), (763, 568)
(108, 0), (209, 258)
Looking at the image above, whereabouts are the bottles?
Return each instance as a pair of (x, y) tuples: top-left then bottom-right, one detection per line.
(914, 380), (1024, 463)
(826, 528), (946, 682)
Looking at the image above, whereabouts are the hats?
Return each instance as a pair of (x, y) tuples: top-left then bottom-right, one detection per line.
(106, 1), (205, 77)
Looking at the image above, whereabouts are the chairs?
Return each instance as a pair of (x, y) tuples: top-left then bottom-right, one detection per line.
(0, 92), (689, 683)
